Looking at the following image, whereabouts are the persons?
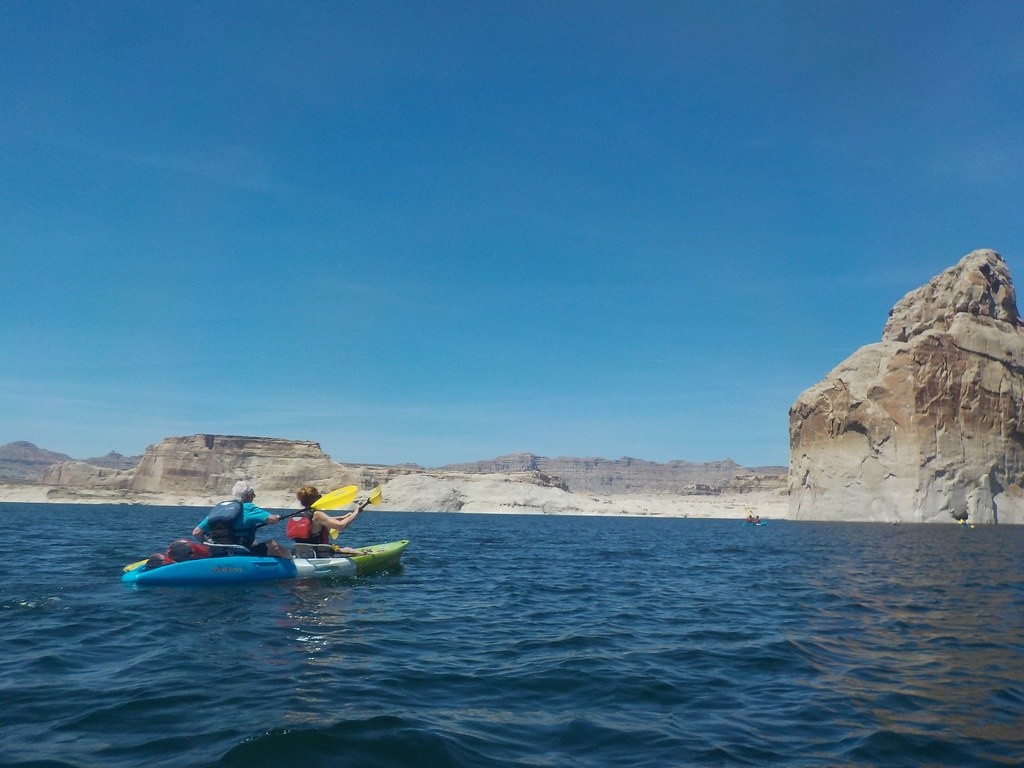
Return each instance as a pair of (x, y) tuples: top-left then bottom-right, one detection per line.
(287, 486), (369, 556)
(746, 515), (753, 523)
(192, 481), (289, 556)
(754, 516), (760, 524)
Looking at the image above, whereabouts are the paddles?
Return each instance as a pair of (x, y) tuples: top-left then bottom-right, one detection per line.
(329, 486), (383, 540)
(124, 485), (359, 572)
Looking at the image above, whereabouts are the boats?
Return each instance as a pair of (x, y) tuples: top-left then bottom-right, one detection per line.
(120, 538), (410, 582)
